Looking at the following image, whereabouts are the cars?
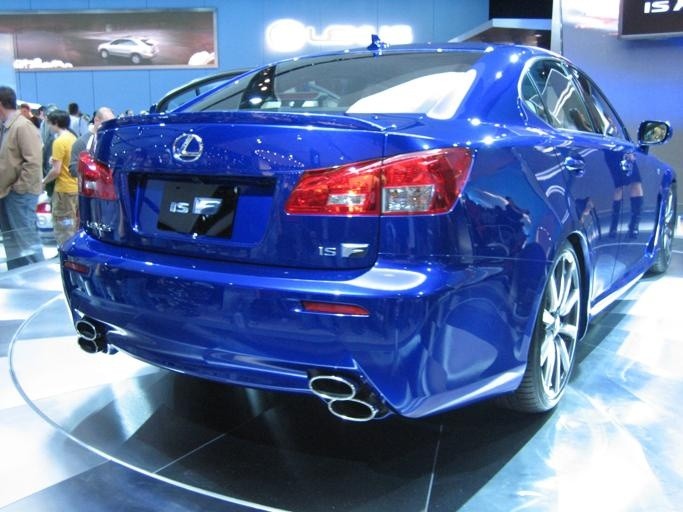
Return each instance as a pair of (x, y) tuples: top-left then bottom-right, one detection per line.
(61, 31), (678, 425)
(96, 33), (160, 64)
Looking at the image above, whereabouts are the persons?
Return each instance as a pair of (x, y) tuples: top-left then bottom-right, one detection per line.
(17, 103), (58, 197)
(66, 102), (96, 140)
(0, 85), (46, 270)
(41, 108), (81, 261)
(124, 109), (134, 117)
(68, 107), (115, 232)
(608, 141), (646, 246)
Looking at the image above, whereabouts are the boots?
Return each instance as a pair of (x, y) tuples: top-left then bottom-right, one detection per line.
(629, 196), (643, 236)
(609, 201), (621, 238)
